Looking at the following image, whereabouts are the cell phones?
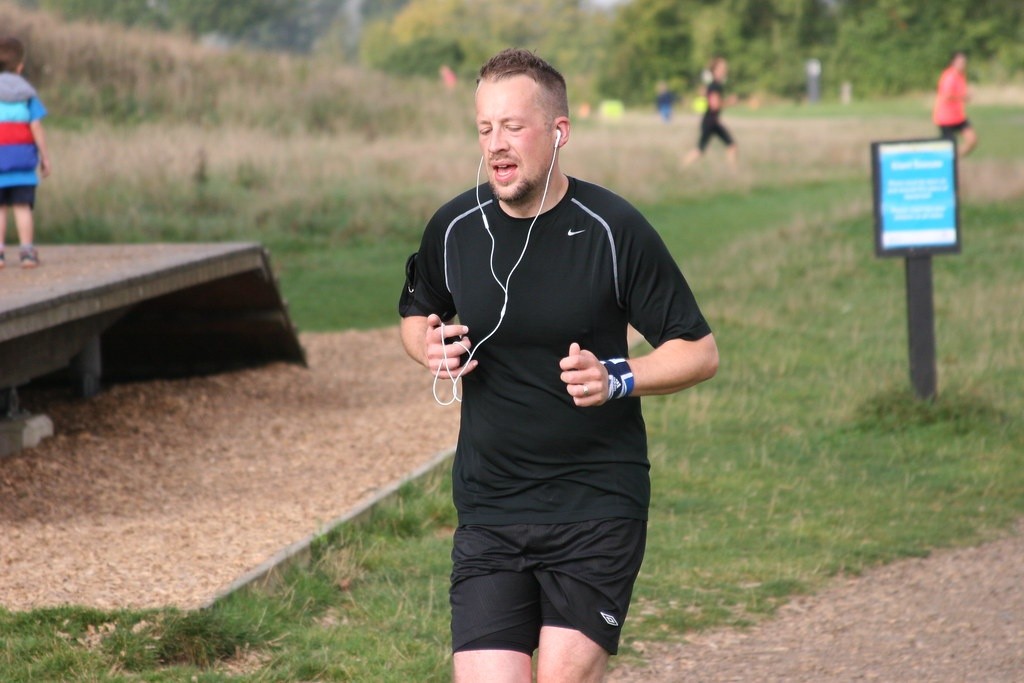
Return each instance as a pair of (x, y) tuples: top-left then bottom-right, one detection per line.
(433, 325), (469, 366)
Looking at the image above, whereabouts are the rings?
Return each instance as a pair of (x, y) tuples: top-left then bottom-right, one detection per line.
(583, 383), (592, 397)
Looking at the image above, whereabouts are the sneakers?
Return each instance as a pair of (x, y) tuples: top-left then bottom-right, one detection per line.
(17, 243), (40, 267)
(0, 245), (5, 269)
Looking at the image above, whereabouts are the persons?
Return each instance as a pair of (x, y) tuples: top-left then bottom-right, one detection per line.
(395, 49), (721, 683)
(677, 53), (743, 172)
(440, 43), (856, 123)
(932, 48), (982, 161)
(1, 36), (51, 269)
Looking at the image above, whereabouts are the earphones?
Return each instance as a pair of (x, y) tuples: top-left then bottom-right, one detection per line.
(554, 129), (561, 148)
